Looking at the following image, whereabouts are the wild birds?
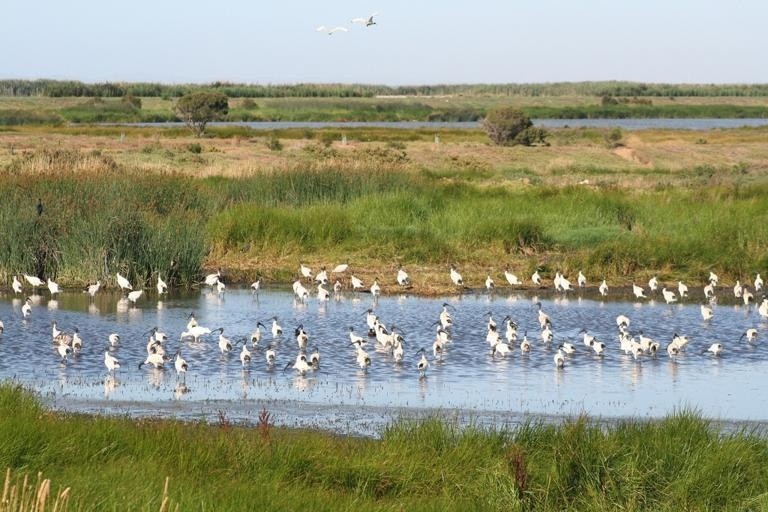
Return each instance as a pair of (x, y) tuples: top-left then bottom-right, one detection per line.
(349, 9), (390, 27)
(0, 259), (767, 379)
(313, 26), (355, 36)
